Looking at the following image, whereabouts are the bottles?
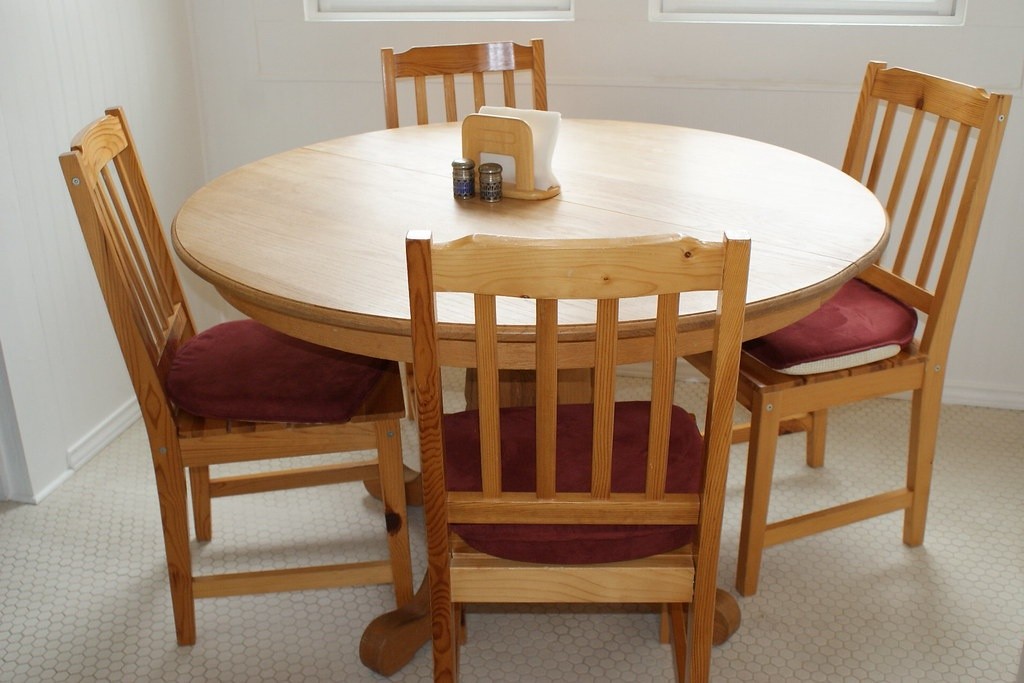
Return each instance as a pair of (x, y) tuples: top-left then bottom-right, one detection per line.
(452, 158), (475, 198)
(479, 162), (503, 203)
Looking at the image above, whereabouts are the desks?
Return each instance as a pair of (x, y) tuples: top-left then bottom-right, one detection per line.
(173, 116), (891, 677)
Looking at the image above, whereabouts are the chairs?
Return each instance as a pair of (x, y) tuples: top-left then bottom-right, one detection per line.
(402, 226), (752, 681)
(679, 63), (1012, 595)
(381, 36), (552, 421)
(58, 109), (415, 649)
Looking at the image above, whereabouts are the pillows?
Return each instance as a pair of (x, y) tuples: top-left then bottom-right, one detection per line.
(170, 315), (377, 425)
(434, 399), (711, 564)
(743, 277), (919, 374)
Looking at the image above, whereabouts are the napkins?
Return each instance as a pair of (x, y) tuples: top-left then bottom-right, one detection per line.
(473, 104), (563, 191)
